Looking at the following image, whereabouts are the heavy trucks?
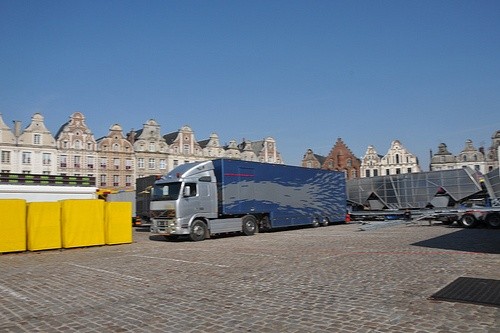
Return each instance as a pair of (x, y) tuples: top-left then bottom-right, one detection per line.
(148, 157), (347, 241)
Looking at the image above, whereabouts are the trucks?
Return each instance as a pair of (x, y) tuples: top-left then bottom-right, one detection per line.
(433, 207), (500, 229)
